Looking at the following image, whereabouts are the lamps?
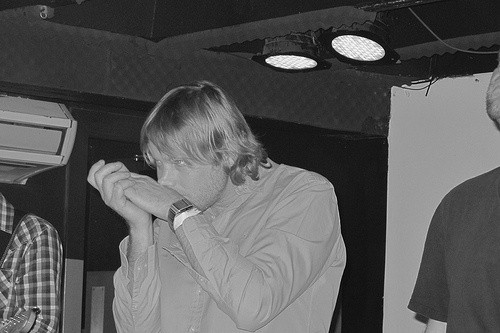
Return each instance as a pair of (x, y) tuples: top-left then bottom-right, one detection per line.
(324, 19), (402, 69)
(250, 30), (331, 75)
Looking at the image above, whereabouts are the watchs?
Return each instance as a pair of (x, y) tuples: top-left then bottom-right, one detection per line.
(168, 197), (194, 233)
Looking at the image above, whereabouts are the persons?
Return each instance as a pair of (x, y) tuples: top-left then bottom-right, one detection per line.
(84, 79), (346, 332)
(407, 62), (500, 332)
(0, 191), (65, 333)
